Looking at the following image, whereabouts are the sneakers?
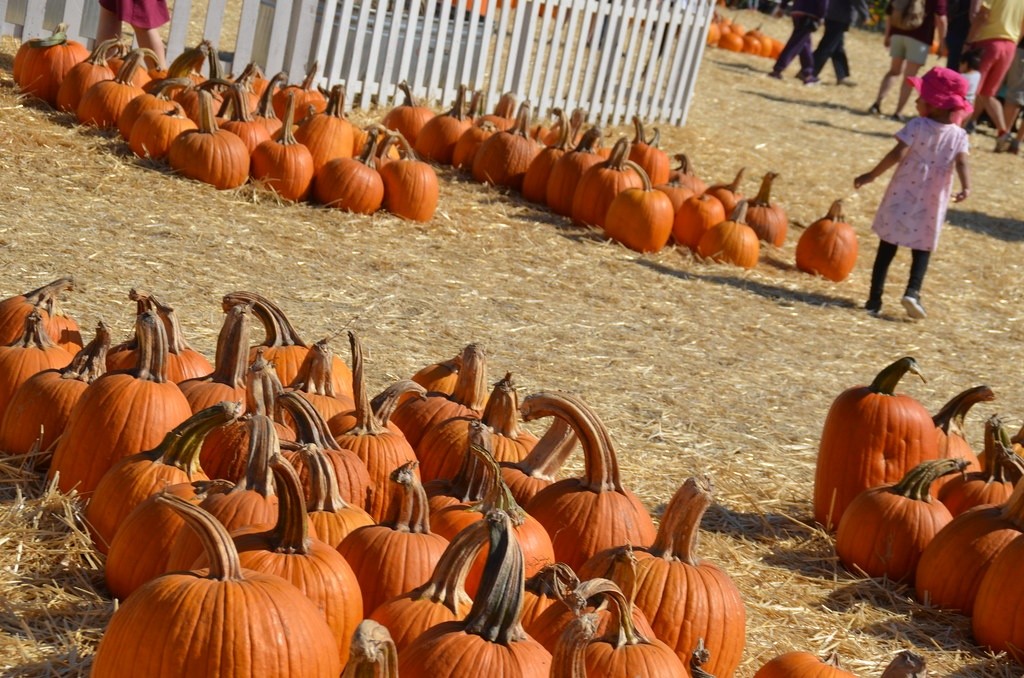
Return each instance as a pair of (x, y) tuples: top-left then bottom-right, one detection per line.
(901, 290), (928, 320)
(865, 300), (883, 315)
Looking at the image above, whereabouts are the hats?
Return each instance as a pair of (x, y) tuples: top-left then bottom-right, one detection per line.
(906, 66), (975, 117)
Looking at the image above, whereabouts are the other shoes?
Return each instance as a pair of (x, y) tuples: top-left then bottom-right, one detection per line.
(1008, 139), (1020, 154)
(870, 102), (885, 119)
(767, 69), (858, 87)
(994, 131), (1012, 154)
(891, 113), (906, 123)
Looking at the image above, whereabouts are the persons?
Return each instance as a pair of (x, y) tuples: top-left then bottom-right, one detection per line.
(854, 66), (975, 322)
(95, 0), (172, 69)
(722, 0), (1024, 155)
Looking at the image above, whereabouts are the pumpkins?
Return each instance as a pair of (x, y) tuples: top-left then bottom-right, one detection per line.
(815, 358), (1024, 667)
(13, 22), (786, 271)
(0, 276), (861, 678)
(797, 198), (857, 282)
(928, 38), (947, 57)
(706, 10), (785, 58)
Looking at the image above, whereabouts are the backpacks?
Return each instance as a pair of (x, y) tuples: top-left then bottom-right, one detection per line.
(891, 0), (925, 30)
